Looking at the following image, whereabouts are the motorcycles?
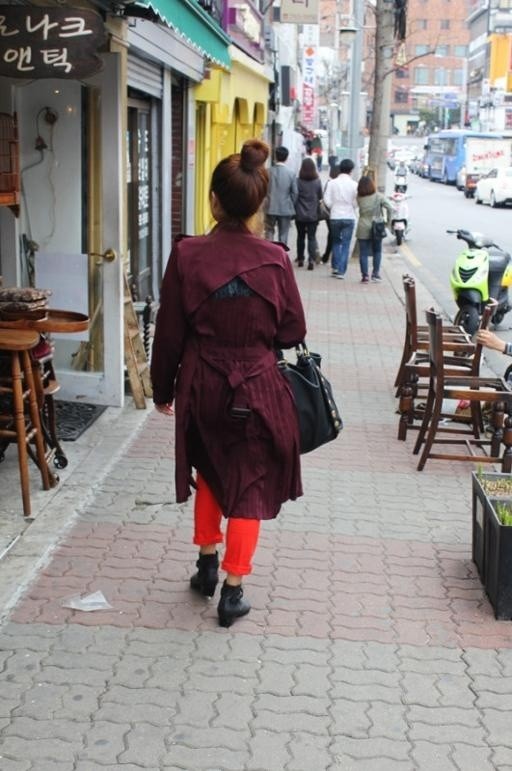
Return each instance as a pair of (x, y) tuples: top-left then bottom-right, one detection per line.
(446, 227), (512, 335)
(388, 192), (412, 245)
(396, 173), (408, 193)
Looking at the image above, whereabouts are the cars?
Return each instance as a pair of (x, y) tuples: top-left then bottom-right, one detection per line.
(387, 130), (512, 208)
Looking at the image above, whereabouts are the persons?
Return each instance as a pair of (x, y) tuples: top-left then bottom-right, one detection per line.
(150, 139), (306, 628)
(263, 147), (409, 283)
(476, 328), (511, 356)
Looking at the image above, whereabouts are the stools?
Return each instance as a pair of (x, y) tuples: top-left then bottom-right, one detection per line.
(0, 328), (52, 517)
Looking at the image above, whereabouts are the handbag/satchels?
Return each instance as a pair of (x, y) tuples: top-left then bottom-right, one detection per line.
(276, 352), (343, 454)
(372, 221), (387, 240)
(318, 198), (329, 220)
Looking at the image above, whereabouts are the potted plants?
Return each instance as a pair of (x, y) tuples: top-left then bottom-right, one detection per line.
(471, 462), (512, 620)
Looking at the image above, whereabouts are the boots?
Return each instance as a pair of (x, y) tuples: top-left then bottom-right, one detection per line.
(218, 580), (250, 627)
(191, 551), (219, 597)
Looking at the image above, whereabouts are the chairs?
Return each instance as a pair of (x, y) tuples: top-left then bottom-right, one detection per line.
(394, 273), (512, 473)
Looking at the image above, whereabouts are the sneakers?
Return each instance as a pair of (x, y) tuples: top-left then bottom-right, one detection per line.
(298, 255), (383, 284)
